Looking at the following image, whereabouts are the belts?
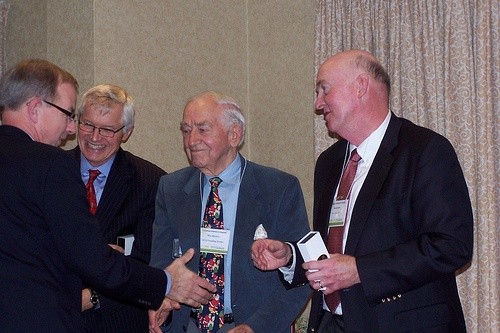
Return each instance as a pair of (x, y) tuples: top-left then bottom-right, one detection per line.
(192, 312), (234, 324)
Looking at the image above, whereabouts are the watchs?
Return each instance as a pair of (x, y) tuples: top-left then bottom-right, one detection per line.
(88, 286), (99, 307)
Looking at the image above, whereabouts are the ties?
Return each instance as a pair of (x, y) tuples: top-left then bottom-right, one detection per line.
(197, 177), (225, 333)
(85, 170), (101, 215)
(324, 148), (362, 314)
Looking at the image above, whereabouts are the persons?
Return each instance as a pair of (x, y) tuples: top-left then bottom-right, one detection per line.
(146, 93), (315, 333)
(61, 85), (167, 333)
(251, 50), (473, 333)
(0, 59), (218, 333)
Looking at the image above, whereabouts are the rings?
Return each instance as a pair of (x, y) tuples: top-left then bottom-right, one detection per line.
(319, 281), (323, 288)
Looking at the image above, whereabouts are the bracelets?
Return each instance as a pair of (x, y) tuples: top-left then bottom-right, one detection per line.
(284, 244), (294, 267)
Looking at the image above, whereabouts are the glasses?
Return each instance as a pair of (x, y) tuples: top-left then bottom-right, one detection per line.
(26, 99), (79, 121)
(79, 121), (126, 138)
